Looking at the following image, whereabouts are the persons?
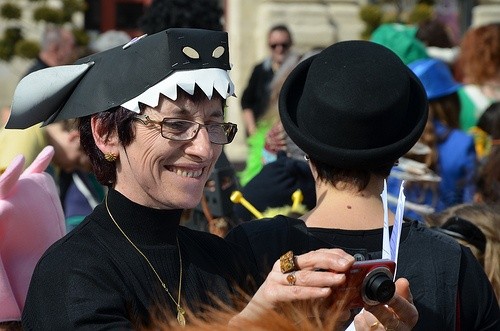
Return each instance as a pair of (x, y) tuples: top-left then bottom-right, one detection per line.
(0, 0), (500, 331)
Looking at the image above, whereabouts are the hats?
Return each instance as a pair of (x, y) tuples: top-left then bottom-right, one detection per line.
(4, 28), (237, 129)
(278, 39), (429, 168)
(405, 59), (463, 99)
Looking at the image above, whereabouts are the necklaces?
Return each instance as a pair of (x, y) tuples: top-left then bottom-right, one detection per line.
(105, 195), (186, 328)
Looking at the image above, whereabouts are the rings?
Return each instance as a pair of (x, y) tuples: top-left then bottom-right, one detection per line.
(287, 271), (295, 286)
(385, 317), (399, 330)
(280, 251), (296, 274)
(369, 321), (382, 331)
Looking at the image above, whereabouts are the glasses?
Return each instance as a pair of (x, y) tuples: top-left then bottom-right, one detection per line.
(132, 114), (237, 144)
(270, 43), (290, 49)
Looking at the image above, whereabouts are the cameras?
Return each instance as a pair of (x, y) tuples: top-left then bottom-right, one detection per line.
(322, 259), (396, 309)
